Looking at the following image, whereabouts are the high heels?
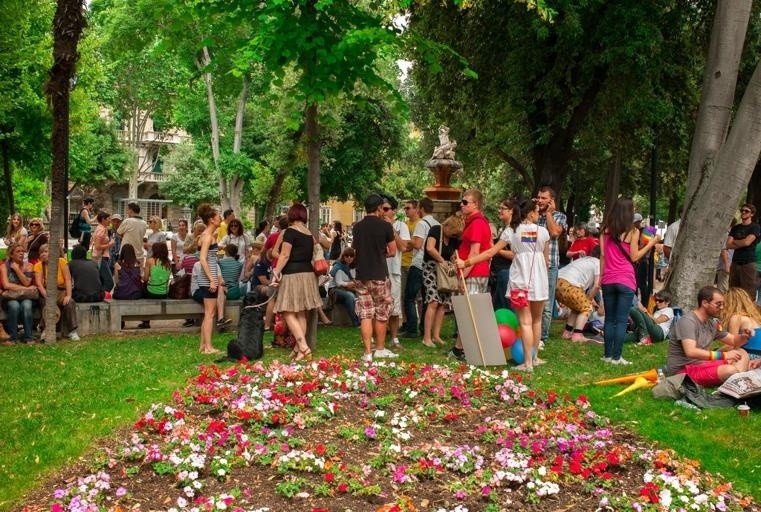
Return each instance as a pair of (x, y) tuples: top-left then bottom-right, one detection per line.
(289, 347), (312, 361)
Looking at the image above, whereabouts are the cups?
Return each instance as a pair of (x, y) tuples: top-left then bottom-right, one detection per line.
(738, 405), (751, 417)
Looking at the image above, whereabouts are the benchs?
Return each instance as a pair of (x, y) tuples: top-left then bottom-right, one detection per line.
(73, 297), (243, 337)
(317, 299), (353, 328)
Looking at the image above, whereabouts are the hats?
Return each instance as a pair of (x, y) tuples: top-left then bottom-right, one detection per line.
(633, 213), (642, 223)
(111, 214), (122, 221)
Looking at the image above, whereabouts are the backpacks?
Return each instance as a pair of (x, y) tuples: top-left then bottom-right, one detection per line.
(70, 208), (86, 238)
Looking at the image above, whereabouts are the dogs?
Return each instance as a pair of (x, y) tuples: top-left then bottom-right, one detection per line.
(212, 291), (269, 363)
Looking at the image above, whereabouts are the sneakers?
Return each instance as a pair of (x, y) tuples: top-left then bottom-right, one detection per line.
(422, 337), (445, 347)
(510, 289), (528, 308)
(216, 319), (232, 326)
(562, 330), (604, 343)
(68, 331), (80, 341)
(638, 337), (652, 346)
(448, 347), (465, 360)
(604, 356), (632, 365)
(436, 262), (459, 292)
(361, 348), (399, 361)
(391, 338), (401, 348)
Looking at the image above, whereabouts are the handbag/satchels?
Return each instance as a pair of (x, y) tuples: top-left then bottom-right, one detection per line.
(2, 289), (39, 300)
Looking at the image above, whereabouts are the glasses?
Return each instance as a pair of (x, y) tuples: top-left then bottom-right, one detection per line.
(462, 200), (467, 205)
(655, 299), (663, 303)
(383, 207), (391, 211)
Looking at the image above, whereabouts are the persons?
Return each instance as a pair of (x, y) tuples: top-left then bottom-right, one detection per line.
(0, 181), (761, 411)
